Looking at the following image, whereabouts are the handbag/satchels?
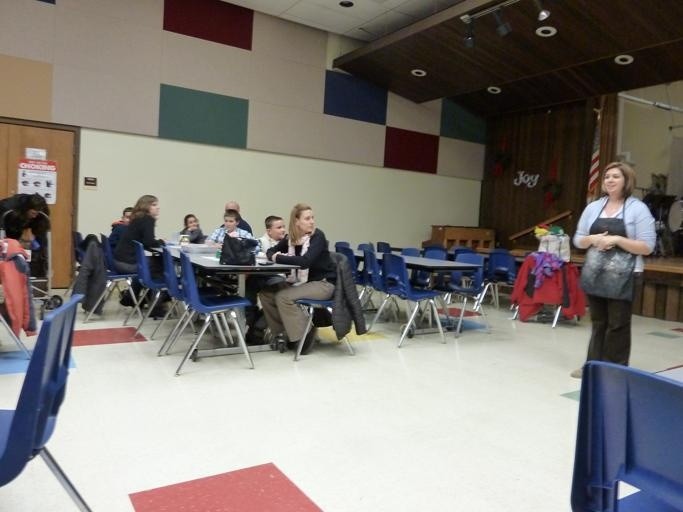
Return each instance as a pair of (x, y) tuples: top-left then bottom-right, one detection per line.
(220, 232), (258, 266)
(538, 234), (571, 263)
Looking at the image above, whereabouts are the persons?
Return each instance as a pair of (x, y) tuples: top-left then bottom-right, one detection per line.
(572, 161), (658, 365)
(0, 192), (50, 297)
(179, 213), (205, 245)
(220, 200), (254, 238)
(258, 202), (338, 354)
(112, 195), (172, 318)
(108, 206), (144, 307)
(205, 208), (256, 246)
(245, 214), (287, 344)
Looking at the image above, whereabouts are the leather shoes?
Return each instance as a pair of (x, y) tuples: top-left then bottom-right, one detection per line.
(301, 325), (316, 356)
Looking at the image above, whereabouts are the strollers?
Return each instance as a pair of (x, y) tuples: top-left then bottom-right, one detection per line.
(0, 211), (62, 310)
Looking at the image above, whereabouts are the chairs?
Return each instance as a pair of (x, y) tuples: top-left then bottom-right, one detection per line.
(569, 358), (683, 512)
(0, 291), (97, 512)
